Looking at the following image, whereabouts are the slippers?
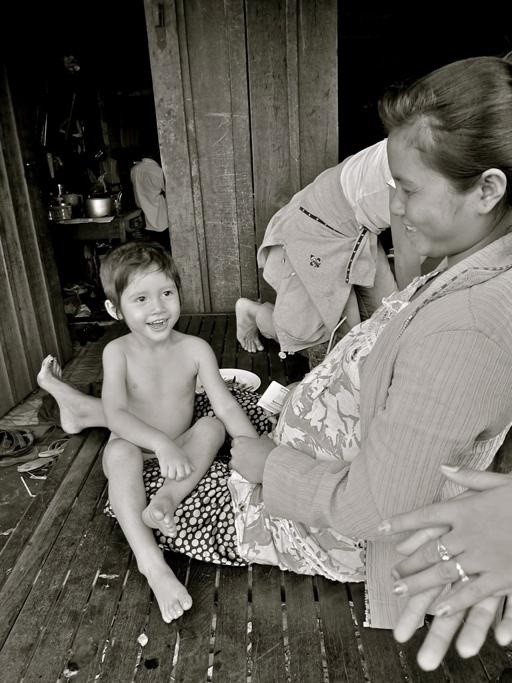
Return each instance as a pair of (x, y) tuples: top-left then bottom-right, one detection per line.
(0, 424), (71, 480)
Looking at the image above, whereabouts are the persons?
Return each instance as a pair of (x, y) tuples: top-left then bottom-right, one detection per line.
(33, 52), (511, 632)
(230, 134), (448, 358)
(369, 457), (512, 671)
(128, 152), (172, 256)
(95, 239), (265, 624)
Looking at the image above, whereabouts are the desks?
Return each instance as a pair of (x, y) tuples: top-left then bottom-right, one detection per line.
(52, 210), (143, 245)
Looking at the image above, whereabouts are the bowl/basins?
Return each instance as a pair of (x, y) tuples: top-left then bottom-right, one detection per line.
(81, 197), (111, 219)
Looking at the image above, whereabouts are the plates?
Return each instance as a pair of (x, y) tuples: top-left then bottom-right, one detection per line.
(195, 365), (263, 396)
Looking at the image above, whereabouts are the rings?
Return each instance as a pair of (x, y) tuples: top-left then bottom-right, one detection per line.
(436, 536), (453, 564)
(453, 559), (471, 583)
